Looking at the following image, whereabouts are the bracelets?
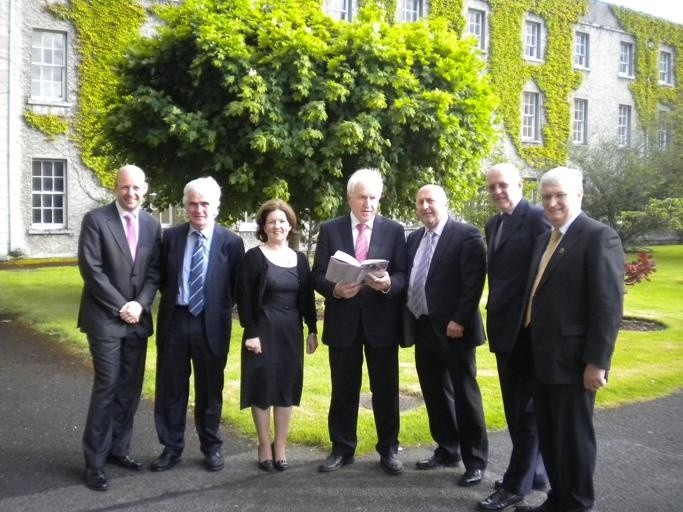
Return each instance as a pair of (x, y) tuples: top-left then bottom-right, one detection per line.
(310, 332), (316, 336)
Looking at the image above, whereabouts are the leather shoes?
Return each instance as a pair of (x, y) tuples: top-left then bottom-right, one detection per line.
(416, 455), (459, 470)
(203, 450), (225, 471)
(457, 467), (484, 487)
(476, 486), (594, 512)
(380, 453), (405, 475)
(109, 452), (144, 471)
(494, 474), (549, 492)
(257, 445), (274, 472)
(270, 441), (289, 471)
(318, 452), (354, 472)
(150, 446), (182, 471)
(84, 466), (109, 491)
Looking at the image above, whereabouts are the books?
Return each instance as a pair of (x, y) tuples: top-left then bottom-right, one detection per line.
(325, 250), (390, 286)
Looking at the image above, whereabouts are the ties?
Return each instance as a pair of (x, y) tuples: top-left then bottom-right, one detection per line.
(406, 230), (433, 320)
(523, 229), (563, 330)
(123, 213), (136, 262)
(354, 224), (369, 264)
(186, 231), (206, 317)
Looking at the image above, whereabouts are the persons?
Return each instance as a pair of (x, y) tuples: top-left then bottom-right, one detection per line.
(76, 164), (162, 491)
(150, 176), (246, 471)
(477, 162), (552, 512)
(405, 183), (489, 487)
(310, 168), (409, 475)
(511, 167), (625, 512)
(237, 198), (319, 470)
